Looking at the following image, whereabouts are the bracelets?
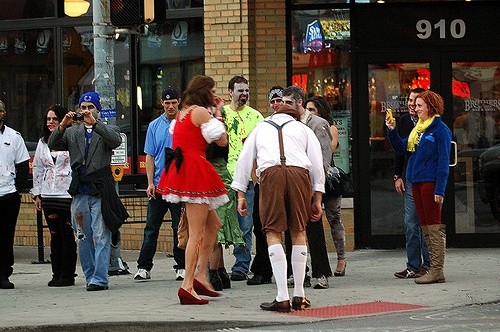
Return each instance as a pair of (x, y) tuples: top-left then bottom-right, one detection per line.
(238, 198), (246, 201)
(94, 119), (100, 127)
(59, 124), (65, 127)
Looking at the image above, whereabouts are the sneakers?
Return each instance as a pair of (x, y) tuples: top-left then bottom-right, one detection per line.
(246, 274), (272, 285)
(287, 274), (311, 288)
(134, 268), (150, 280)
(313, 274), (329, 289)
(231, 271), (246, 281)
(394, 267), (416, 278)
(175, 269), (185, 281)
(415, 267), (430, 278)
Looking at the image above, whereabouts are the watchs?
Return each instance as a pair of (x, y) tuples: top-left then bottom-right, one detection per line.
(393, 175), (400, 181)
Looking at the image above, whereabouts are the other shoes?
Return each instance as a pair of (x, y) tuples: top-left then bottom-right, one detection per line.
(0, 278), (14, 289)
(86, 284), (108, 291)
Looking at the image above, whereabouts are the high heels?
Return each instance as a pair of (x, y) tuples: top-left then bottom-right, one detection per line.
(333, 262), (347, 277)
(178, 287), (209, 306)
(218, 268), (231, 289)
(209, 270), (222, 291)
(192, 279), (220, 297)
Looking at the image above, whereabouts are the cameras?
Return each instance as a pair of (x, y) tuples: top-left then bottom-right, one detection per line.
(72, 114), (84, 120)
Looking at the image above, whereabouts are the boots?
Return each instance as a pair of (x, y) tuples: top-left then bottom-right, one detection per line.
(47, 254), (61, 287)
(54, 253), (78, 287)
(414, 226), (446, 284)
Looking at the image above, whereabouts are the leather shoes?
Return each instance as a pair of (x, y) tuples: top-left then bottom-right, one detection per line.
(292, 297), (311, 310)
(260, 299), (291, 312)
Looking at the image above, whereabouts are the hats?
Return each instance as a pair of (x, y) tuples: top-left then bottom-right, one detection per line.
(162, 85), (178, 100)
(78, 92), (101, 111)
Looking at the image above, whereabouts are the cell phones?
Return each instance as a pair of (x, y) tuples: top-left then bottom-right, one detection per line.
(386, 109), (395, 123)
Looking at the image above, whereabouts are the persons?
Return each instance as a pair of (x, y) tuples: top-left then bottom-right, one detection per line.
(135, 76), (347, 312)
(385, 87), (450, 283)
(46, 92), (132, 292)
(453, 98), (497, 206)
(33, 105), (78, 287)
(0, 100), (31, 289)
